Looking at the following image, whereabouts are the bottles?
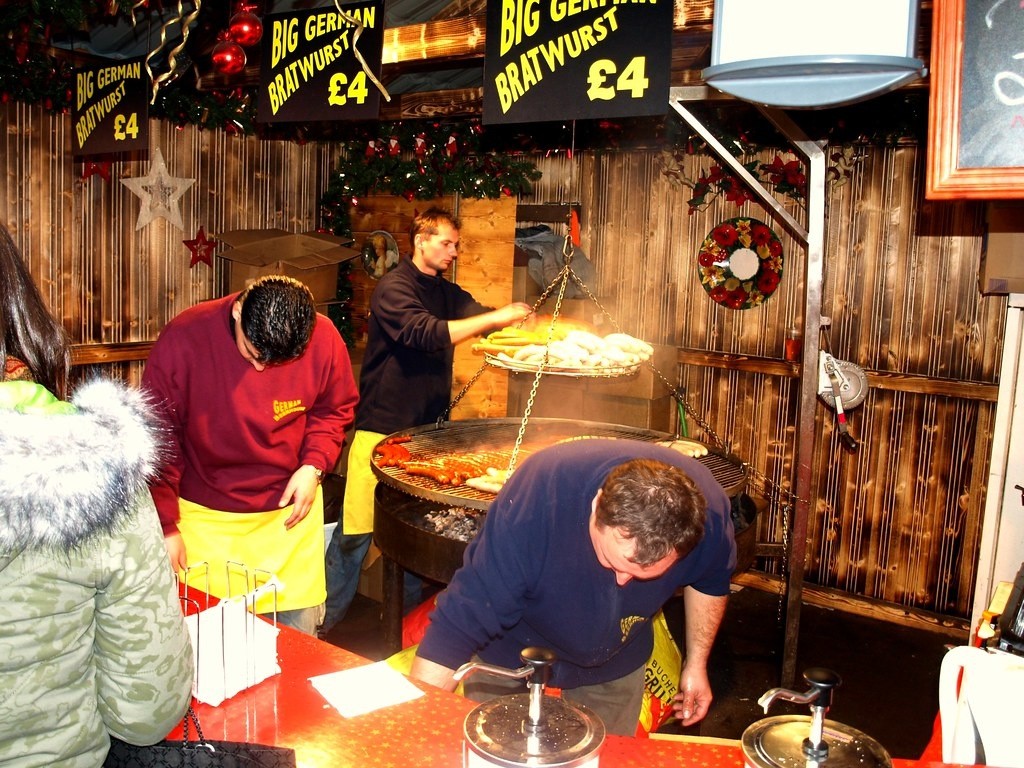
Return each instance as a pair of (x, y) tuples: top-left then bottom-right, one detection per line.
(785, 327), (802, 362)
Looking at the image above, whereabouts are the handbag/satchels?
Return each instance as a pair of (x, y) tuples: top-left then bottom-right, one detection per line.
(101, 704), (296, 768)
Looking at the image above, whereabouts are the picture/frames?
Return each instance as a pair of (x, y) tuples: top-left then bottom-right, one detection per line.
(924, 0), (1024, 201)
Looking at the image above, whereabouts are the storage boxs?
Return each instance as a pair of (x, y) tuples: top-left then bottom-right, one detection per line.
(512, 267), (541, 303)
(528, 295), (615, 339)
(514, 245), (529, 266)
(315, 299), (348, 316)
(214, 228), (363, 302)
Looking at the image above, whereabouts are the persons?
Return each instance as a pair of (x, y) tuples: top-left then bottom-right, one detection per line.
(409, 439), (737, 737)
(137, 276), (362, 640)
(314, 206), (532, 642)
(0, 221), (194, 768)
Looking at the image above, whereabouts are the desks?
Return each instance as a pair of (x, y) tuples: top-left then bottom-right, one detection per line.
(164, 582), (998, 768)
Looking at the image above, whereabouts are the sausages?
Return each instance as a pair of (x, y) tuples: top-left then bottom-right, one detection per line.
(658, 440), (708, 459)
(472, 327), (654, 374)
(375, 435), (413, 467)
(404, 450), (535, 495)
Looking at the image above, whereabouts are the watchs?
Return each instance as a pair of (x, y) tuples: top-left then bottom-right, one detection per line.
(315, 468), (326, 484)
